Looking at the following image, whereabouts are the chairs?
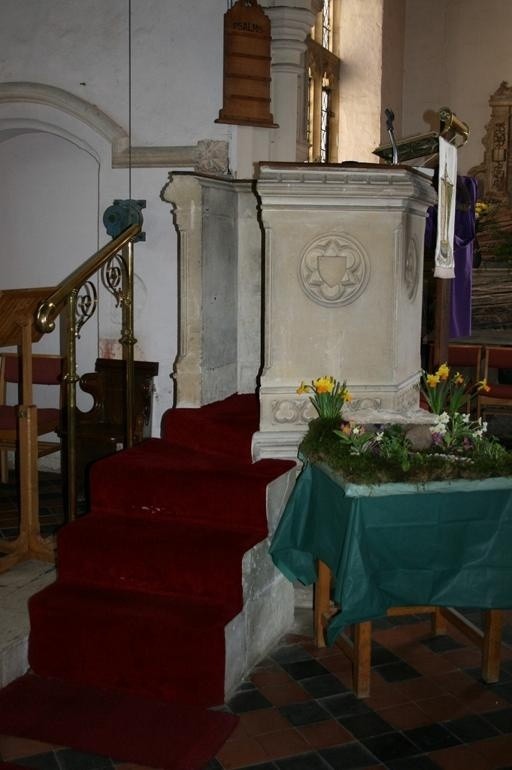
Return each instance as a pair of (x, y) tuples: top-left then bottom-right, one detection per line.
(430, 343), (482, 415)
(476, 346), (512, 431)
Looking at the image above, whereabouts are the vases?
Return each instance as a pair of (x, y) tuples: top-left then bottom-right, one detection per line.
(297, 361), (512, 482)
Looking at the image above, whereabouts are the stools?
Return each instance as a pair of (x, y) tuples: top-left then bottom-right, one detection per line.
(310, 457), (507, 699)
